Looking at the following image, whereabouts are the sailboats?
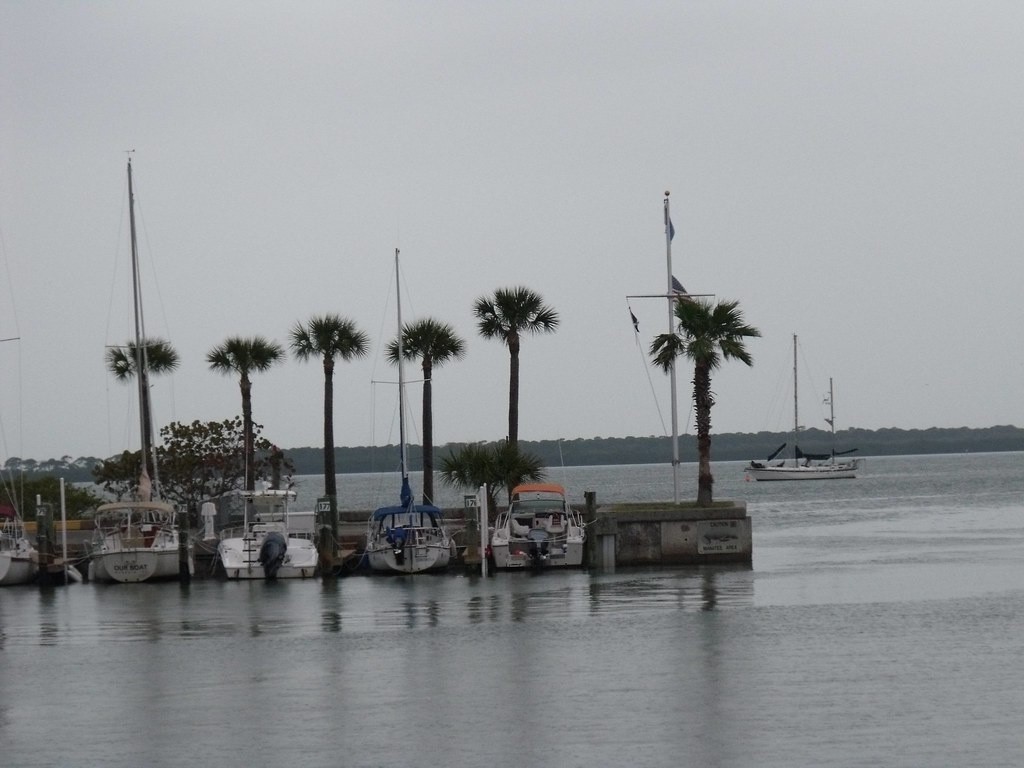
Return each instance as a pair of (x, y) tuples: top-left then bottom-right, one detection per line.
(745, 332), (861, 481)
(359, 248), (460, 575)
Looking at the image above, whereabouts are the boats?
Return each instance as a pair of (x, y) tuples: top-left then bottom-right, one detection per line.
(489, 484), (587, 570)
(194, 420), (321, 580)
(79, 261), (203, 584)
(1, 469), (41, 588)
